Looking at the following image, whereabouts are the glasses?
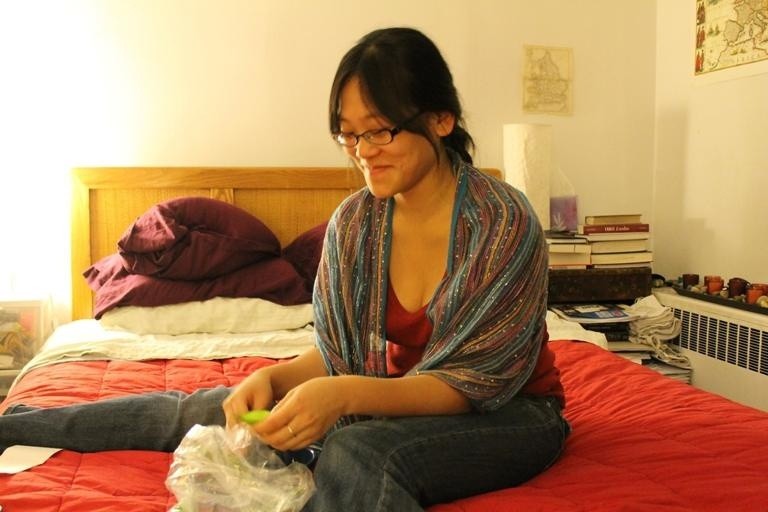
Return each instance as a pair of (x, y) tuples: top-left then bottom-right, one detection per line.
(332, 109), (425, 148)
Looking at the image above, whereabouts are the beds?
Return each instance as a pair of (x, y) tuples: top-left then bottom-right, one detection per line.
(0, 164), (768, 511)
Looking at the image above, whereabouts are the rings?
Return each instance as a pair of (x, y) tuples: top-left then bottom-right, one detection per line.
(287, 422), (297, 437)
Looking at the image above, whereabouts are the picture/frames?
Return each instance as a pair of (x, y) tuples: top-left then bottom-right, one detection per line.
(0, 300), (47, 378)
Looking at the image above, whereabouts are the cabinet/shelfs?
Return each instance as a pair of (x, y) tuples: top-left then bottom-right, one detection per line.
(546, 266), (651, 307)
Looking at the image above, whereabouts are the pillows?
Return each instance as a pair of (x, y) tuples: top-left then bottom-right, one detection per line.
(82, 196), (329, 331)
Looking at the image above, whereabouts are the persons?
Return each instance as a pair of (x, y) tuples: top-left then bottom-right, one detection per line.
(0, 25), (573, 511)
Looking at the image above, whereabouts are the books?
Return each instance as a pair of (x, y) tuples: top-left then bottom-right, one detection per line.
(545, 212), (654, 272)
(550, 300), (693, 386)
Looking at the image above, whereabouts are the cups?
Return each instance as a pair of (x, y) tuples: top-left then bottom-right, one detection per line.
(682, 273), (768, 305)
